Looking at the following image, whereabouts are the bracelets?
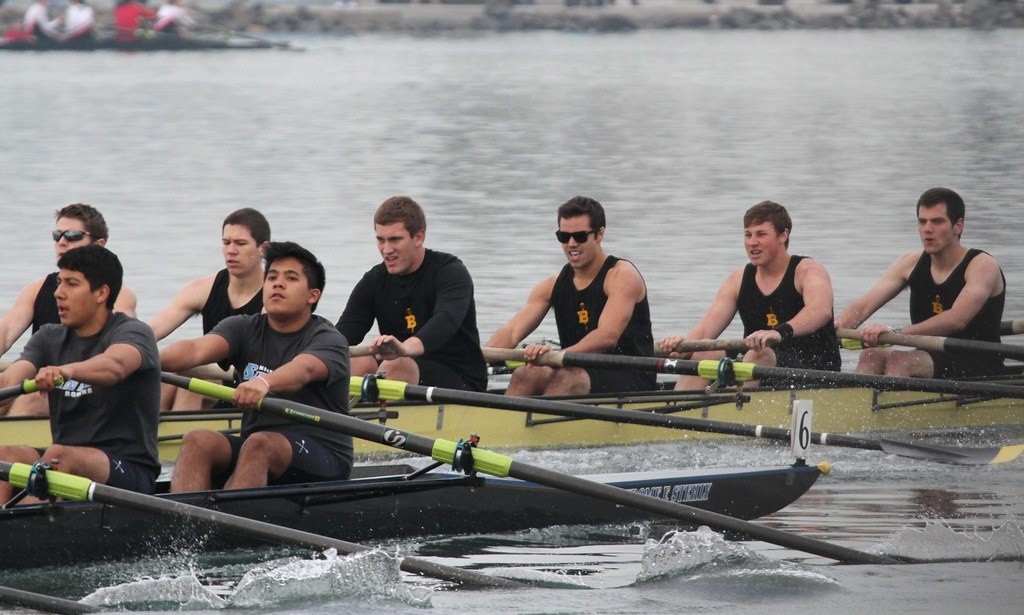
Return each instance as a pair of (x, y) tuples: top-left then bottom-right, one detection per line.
(892, 326), (901, 334)
(773, 322), (793, 343)
(257, 376), (270, 391)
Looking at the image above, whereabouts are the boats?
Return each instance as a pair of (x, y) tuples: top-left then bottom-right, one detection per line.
(0, 463), (829, 570)
(1, 29), (296, 51)
(0, 382), (1024, 450)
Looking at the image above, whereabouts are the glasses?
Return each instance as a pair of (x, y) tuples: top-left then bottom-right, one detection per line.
(555, 228), (600, 243)
(52, 231), (96, 241)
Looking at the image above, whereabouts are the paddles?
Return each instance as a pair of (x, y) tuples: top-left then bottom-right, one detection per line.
(0, 584), (102, 615)
(0, 459), (532, 590)
(158, 369), (906, 566)
(841, 318), (1024, 351)
(651, 338), (772, 356)
(348, 341), (396, 358)
(183, 363), (1024, 466)
(480, 346), (1024, 399)
(836, 327), (1024, 362)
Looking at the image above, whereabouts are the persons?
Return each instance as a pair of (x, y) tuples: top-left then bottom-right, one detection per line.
(655, 200), (842, 390)
(483, 197), (656, 398)
(832, 187), (1007, 379)
(332, 196), (489, 392)
(146, 207), (271, 413)
(0, 245), (162, 506)
(156, 238), (354, 493)
(0, 203), (137, 417)
